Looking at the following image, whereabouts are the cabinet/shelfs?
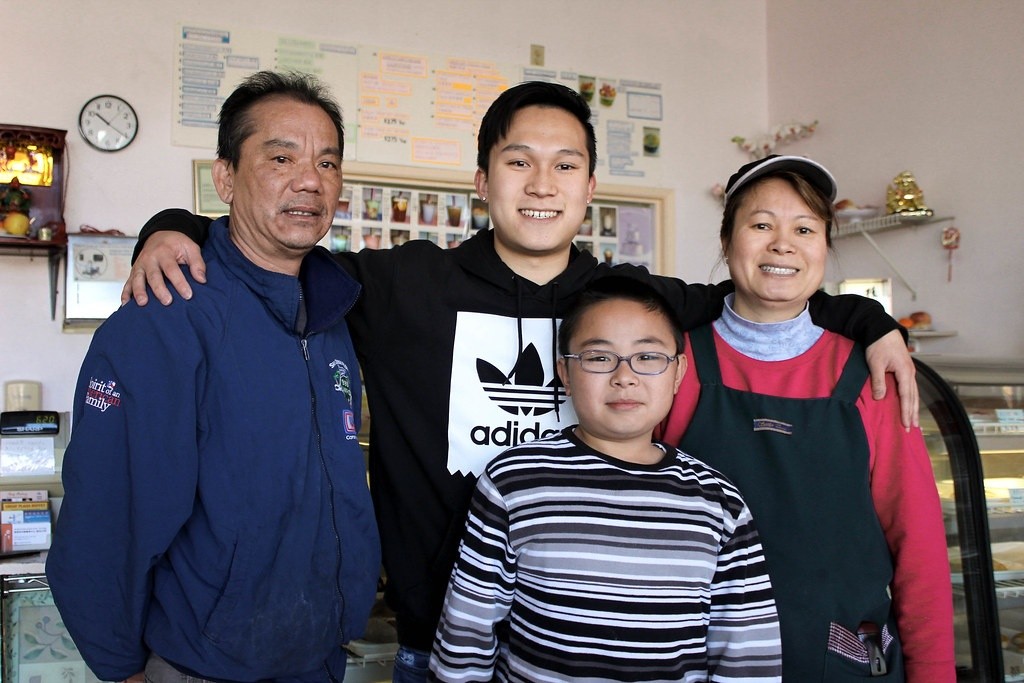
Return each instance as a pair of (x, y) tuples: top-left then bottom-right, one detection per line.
(914, 352), (1024, 683)
(832, 215), (957, 338)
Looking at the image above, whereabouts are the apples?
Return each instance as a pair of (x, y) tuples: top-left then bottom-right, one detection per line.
(899, 312), (931, 330)
(0, 212), (29, 235)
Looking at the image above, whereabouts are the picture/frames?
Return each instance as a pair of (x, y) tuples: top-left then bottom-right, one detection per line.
(311, 161), (674, 277)
(192, 159), (231, 220)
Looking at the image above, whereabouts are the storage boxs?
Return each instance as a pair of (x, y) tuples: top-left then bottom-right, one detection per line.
(0, 501), (52, 552)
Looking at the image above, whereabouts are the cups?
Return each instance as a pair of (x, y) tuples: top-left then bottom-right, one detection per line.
(330, 194), (488, 250)
(6, 381), (40, 411)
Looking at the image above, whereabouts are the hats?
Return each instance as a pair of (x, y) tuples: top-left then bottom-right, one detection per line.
(723, 153), (838, 205)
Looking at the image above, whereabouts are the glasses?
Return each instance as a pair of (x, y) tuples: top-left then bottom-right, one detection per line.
(556, 351), (679, 376)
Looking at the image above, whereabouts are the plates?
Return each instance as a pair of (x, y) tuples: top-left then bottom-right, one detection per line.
(835, 208), (879, 223)
(0, 234), (31, 238)
(906, 329), (935, 332)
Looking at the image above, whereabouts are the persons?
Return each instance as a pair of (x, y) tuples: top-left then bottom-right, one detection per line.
(46, 71), (380, 683)
(426, 277), (781, 683)
(121, 81), (922, 683)
(652, 155), (956, 683)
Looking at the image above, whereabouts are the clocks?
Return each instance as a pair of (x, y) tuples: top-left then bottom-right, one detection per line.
(78, 95), (139, 152)
